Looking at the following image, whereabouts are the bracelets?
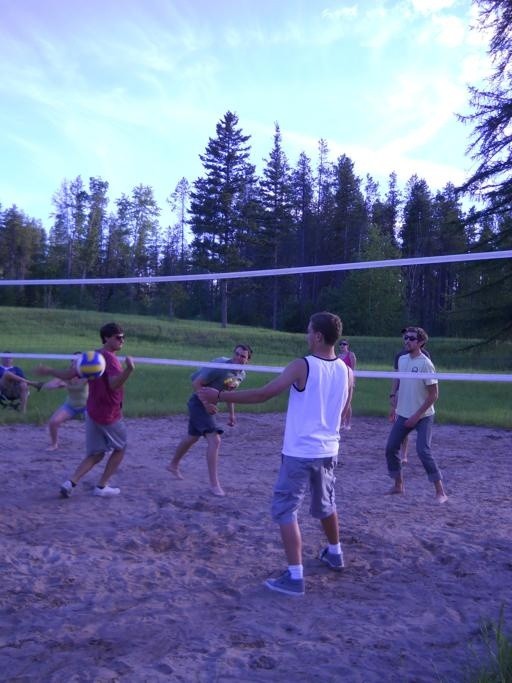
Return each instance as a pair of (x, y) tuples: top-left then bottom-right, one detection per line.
(218, 389), (224, 400)
(390, 394), (395, 397)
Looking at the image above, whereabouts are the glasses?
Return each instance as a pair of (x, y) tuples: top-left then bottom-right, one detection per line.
(404, 334), (418, 342)
(113, 335), (125, 341)
(339, 343), (348, 346)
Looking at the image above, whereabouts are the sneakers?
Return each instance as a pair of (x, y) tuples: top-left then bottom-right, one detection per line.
(317, 545), (345, 572)
(92, 483), (121, 498)
(60, 480), (73, 499)
(263, 568), (306, 597)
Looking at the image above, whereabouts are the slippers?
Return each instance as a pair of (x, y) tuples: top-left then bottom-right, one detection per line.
(37, 382), (44, 393)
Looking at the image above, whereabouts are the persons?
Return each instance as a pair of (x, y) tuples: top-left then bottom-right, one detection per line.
(32, 322), (135, 498)
(43, 351), (89, 452)
(337, 338), (357, 431)
(0, 351), (46, 413)
(385, 326), (448, 505)
(388, 328), (432, 463)
(196, 310), (355, 597)
(166, 343), (253, 498)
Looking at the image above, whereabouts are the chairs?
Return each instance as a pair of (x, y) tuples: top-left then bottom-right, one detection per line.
(0, 390), (31, 413)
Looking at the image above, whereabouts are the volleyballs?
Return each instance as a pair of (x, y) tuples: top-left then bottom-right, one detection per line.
(77, 351), (106, 379)
